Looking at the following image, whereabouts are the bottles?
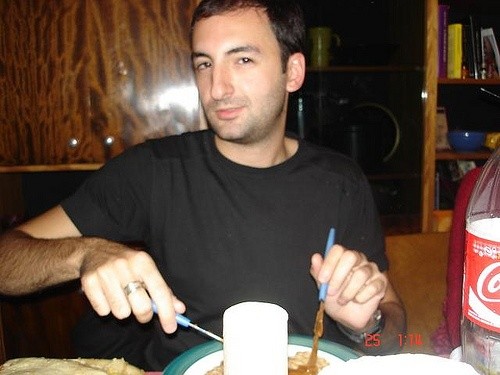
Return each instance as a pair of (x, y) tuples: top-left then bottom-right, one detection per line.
(460, 142), (500, 375)
(480, 64), (487, 80)
(462, 66), (468, 79)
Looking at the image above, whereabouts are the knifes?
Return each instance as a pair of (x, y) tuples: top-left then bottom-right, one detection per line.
(308, 227), (335, 366)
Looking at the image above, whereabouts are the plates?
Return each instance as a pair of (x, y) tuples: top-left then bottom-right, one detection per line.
(162, 335), (361, 375)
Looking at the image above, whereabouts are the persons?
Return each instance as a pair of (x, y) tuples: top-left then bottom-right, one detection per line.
(0, 0), (406, 375)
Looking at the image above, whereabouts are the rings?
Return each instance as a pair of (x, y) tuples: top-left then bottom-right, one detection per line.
(123, 280), (145, 296)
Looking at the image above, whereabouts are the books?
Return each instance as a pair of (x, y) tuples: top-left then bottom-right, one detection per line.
(436, 4), (500, 79)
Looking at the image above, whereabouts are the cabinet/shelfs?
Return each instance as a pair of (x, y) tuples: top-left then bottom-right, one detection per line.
(286, 0), (500, 234)
(0, 0), (208, 361)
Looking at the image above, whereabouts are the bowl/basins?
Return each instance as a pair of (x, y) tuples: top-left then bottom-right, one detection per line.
(447, 132), (484, 152)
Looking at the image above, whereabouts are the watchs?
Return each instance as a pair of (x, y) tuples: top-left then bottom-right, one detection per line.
(336, 310), (386, 344)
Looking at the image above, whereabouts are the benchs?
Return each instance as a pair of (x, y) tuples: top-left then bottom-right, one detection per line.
(383, 234), (450, 356)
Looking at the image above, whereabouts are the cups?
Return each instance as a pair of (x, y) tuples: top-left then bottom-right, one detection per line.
(343, 126), (360, 161)
(487, 65), (493, 78)
(306, 28), (340, 68)
